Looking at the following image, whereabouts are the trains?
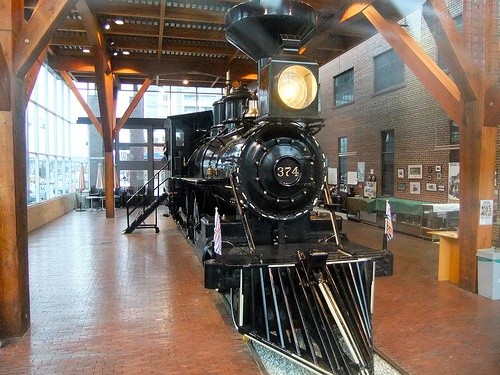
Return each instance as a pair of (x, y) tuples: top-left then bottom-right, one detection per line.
(122, 0), (395, 374)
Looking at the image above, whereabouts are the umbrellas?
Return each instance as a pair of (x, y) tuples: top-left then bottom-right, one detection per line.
(76, 163), (87, 211)
(113, 164), (118, 210)
(96, 162), (106, 210)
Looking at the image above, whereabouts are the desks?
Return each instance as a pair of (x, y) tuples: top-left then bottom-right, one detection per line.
(87, 195), (120, 212)
(346, 195), (460, 284)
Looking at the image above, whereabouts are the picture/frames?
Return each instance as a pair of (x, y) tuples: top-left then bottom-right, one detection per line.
(397, 168), (405, 179)
(410, 182), (420, 194)
(397, 183), (406, 191)
(426, 183), (437, 191)
(408, 165), (422, 179)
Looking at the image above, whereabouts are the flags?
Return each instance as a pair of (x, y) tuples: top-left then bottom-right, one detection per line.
(385, 201), (393, 241)
(212, 212), (222, 254)
